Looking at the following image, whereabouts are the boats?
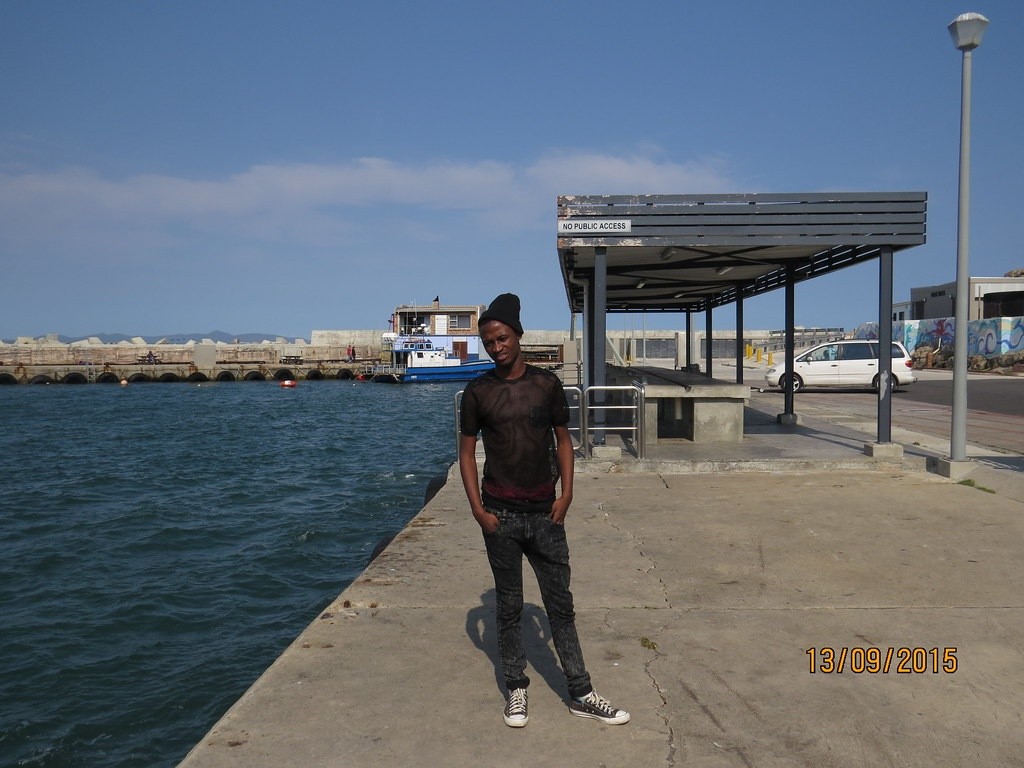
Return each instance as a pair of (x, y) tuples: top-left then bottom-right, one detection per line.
(402, 346), (498, 385)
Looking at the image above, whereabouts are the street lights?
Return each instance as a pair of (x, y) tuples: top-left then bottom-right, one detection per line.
(946, 12), (989, 461)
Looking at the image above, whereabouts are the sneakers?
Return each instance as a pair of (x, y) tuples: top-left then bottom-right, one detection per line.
(569, 692), (631, 725)
(503, 687), (529, 727)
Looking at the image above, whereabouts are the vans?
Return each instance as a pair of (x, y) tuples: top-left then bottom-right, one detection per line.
(765, 338), (917, 392)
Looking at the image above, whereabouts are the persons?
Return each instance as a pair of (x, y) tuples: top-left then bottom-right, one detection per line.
(823, 350), (829, 360)
(459, 307), (634, 727)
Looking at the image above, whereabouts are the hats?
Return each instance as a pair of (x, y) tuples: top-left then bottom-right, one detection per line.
(477, 292), (525, 337)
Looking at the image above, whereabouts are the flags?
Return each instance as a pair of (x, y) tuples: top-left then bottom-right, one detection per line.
(409, 298), (414, 304)
(432, 296), (438, 302)
